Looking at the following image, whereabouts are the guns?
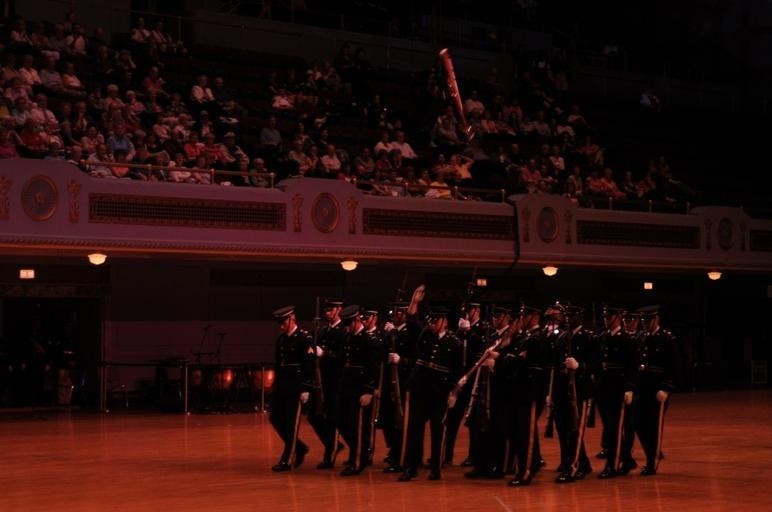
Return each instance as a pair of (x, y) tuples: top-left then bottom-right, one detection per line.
(308, 294), (628, 451)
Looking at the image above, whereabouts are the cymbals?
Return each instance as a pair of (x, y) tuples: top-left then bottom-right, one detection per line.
(191, 351), (216, 356)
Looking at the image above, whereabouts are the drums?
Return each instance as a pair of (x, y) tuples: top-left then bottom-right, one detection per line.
(208, 360), (242, 391)
(245, 360), (276, 392)
(180, 364), (203, 389)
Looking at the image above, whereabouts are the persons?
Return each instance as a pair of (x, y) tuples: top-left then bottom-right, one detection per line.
(270, 297), (685, 480)
(2, 1), (685, 211)
